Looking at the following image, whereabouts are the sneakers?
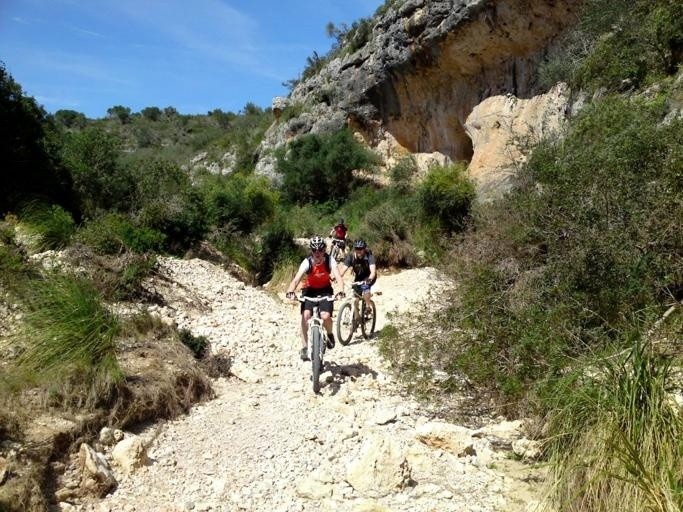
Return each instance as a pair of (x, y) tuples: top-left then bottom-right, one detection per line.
(325, 333), (335, 349)
(300, 346), (309, 360)
(364, 307), (372, 314)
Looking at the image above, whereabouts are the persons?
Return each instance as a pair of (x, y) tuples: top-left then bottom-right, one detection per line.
(337, 239), (378, 325)
(283, 234), (347, 363)
(327, 217), (350, 259)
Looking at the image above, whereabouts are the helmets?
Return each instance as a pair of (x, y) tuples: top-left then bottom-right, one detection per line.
(353, 240), (367, 249)
(339, 219), (344, 224)
(310, 235), (327, 253)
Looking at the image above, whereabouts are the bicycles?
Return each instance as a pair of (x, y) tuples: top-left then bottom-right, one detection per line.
(331, 239), (345, 259)
(334, 280), (383, 345)
(288, 290), (345, 392)
